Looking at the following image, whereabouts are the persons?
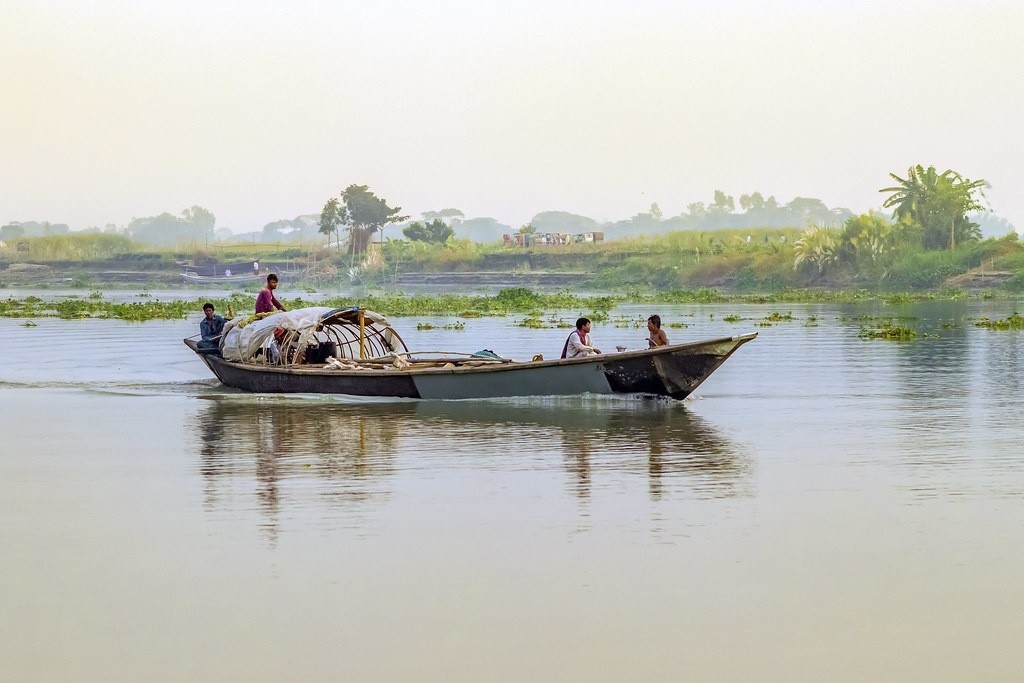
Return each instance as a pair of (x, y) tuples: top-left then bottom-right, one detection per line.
(225, 268), (232, 277)
(561, 317), (602, 359)
(255, 274), (288, 315)
(197, 303), (225, 349)
(253, 262), (259, 276)
(648, 315), (670, 349)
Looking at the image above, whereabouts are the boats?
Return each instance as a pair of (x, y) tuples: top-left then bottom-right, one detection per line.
(182, 307), (758, 404)
